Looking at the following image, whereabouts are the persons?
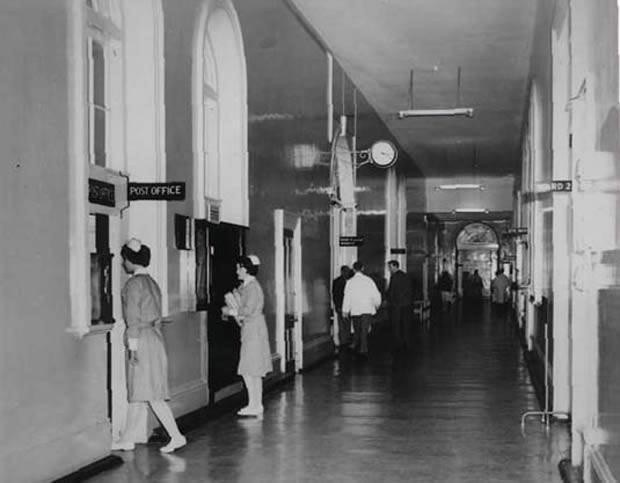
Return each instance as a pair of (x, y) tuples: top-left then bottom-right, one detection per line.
(333, 265), (353, 348)
(219, 254), (274, 417)
(472, 269), (482, 296)
(361, 264), (383, 297)
(499, 268), (510, 304)
(112, 238), (188, 454)
(340, 262), (383, 353)
(386, 260), (413, 352)
(492, 271), (499, 303)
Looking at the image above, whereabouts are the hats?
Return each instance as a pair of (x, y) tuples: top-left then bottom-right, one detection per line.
(247, 254), (261, 266)
(127, 238), (142, 253)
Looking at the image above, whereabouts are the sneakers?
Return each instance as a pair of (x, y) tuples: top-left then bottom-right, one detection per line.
(111, 441), (135, 452)
(237, 406), (264, 416)
(160, 436), (187, 453)
